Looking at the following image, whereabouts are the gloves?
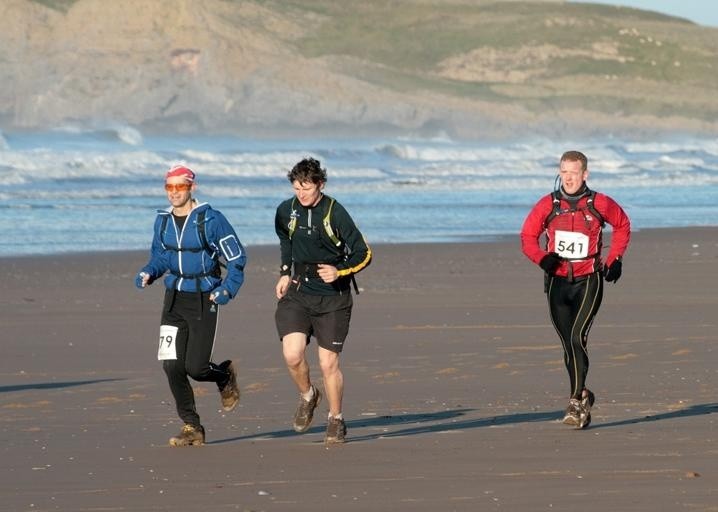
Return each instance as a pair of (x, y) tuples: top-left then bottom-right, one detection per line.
(540, 253), (562, 272)
(209, 287), (229, 304)
(136, 271), (150, 288)
(603, 259), (622, 284)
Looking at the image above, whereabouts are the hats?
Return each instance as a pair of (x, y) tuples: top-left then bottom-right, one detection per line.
(166, 166), (194, 181)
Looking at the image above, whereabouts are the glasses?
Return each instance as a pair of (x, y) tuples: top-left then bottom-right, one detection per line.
(165, 184), (189, 191)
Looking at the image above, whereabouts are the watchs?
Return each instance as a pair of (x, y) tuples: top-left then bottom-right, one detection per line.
(615, 255), (623, 263)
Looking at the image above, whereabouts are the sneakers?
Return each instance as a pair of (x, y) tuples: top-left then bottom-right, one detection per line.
(324, 416), (347, 443)
(293, 385), (322, 432)
(169, 424), (205, 447)
(218, 360), (240, 412)
(563, 390), (594, 429)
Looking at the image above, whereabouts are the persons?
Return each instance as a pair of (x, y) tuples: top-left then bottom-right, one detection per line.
(274, 157), (371, 443)
(134, 160), (246, 450)
(520, 149), (630, 432)
